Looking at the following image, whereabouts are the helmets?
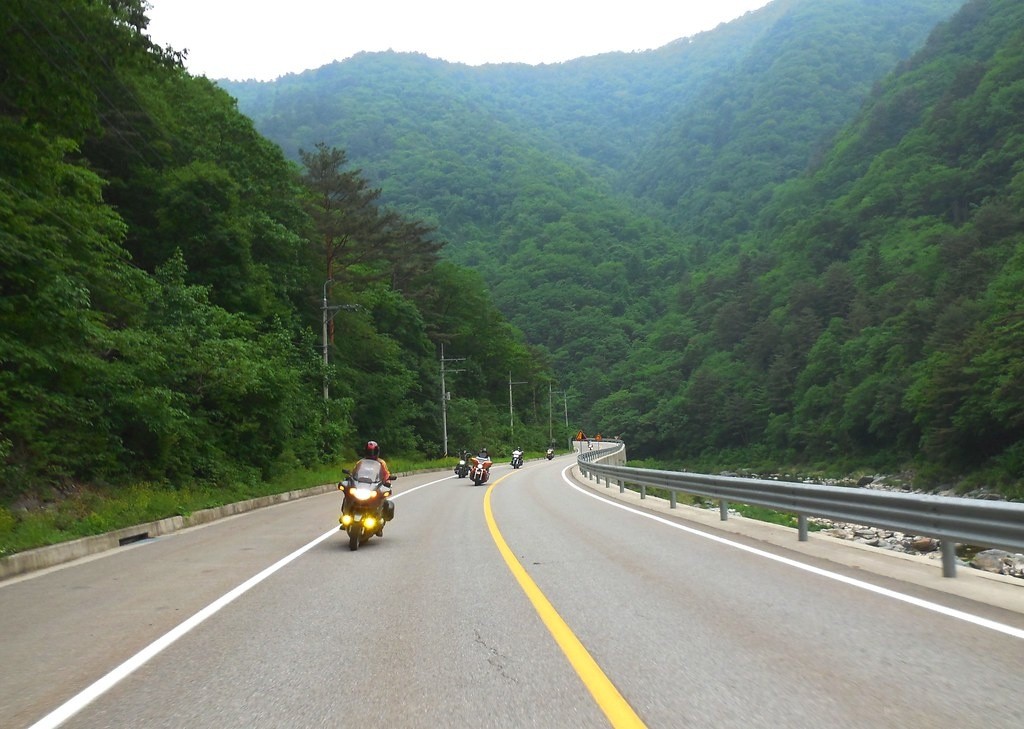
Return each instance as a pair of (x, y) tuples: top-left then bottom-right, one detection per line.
(366, 440), (380, 460)
(463, 449), (467, 453)
(482, 448), (486, 453)
(518, 447), (521, 450)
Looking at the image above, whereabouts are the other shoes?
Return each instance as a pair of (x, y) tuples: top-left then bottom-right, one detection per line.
(340, 524), (345, 530)
(376, 529), (383, 537)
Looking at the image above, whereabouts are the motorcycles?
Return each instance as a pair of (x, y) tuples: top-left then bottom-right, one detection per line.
(511, 450), (524, 469)
(338, 460), (397, 552)
(455, 460), (471, 479)
(468, 452), (493, 487)
(546, 449), (555, 461)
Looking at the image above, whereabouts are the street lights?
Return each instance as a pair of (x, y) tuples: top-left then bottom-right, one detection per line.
(440, 341), (467, 456)
(548, 378), (568, 448)
(563, 389), (577, 428)
(320, 276), (363, 414)
(508, 370), (529, 436)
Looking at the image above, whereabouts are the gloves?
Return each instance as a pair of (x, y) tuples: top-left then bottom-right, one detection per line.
(384, 482), (391, 487)
(346, 477), (349, 481)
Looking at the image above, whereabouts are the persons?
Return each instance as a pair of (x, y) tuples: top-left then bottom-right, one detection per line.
(510, 447), (522, 465)
(473, 448), (491, 472)
(462, 450), (467, 461)
(340, 440), (391, 537)
(548, 446), (554, 451)
